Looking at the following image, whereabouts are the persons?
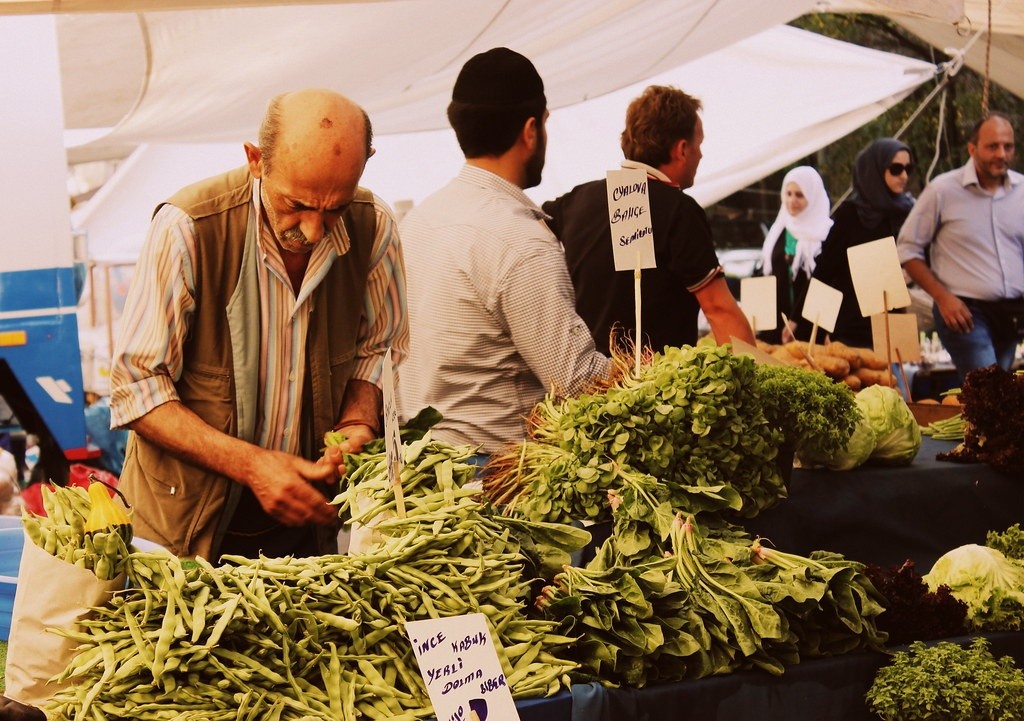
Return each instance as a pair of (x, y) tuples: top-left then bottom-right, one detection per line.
(761, 138), (918, 352)
(108, 90), (409, 561)
(394, 48), (628, 458)
(897, 112), (1024, 387)
(541, 87), (758, 362)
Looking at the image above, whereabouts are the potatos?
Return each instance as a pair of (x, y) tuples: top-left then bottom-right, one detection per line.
(749, 340), (963, 405)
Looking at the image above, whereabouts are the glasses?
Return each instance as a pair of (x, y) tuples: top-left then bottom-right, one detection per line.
(888, 162), (914, 178)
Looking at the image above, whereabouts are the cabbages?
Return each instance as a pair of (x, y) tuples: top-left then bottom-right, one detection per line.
(793, 382), (921, 471)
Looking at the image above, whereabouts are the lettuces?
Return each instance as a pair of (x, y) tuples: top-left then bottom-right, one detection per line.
(935, 363), (1024, 470)
(842, 524), (1024, 648)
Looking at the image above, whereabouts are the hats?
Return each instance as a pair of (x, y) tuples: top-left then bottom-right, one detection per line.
(451, 48), (543, 104)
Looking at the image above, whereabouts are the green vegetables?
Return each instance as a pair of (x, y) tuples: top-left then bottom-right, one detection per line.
(475, 321), (890, 689)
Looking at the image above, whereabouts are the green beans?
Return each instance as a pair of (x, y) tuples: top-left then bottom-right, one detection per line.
(15, 429), (586, 721)
